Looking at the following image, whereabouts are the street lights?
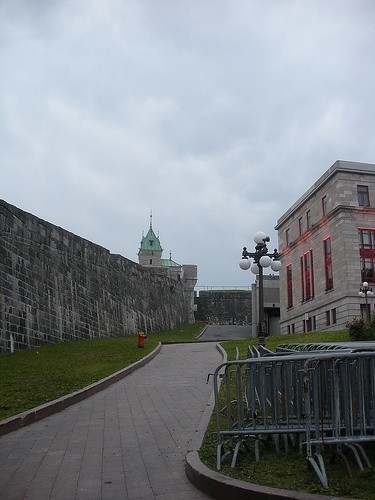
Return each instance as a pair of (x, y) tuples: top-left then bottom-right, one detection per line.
(358, 281), (374, 321)
(239, 232), (283, 340)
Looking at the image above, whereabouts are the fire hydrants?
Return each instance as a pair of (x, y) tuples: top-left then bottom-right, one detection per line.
(138, 330), (147, 347)
